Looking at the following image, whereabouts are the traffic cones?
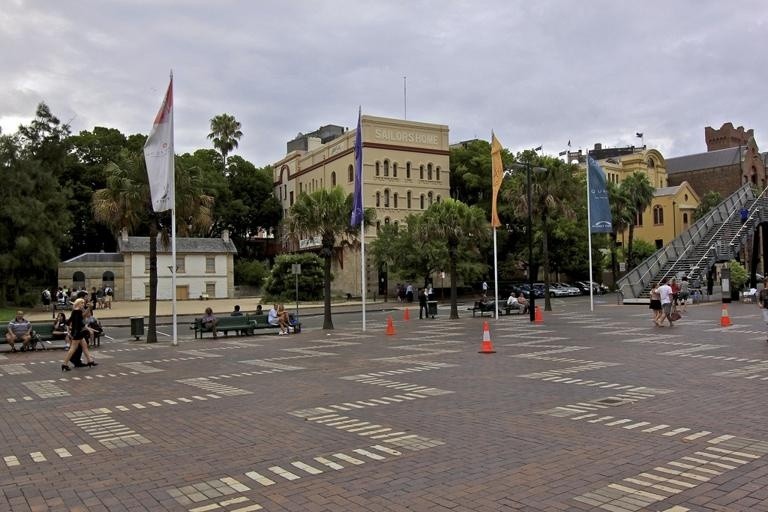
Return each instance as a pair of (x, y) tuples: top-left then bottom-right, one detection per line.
(385, 314), (396, 338)
(533, 305), (545, 324)
(402, 306), (409, 322)
(720, 303), (733, 329)
(476, 320), (497, 355)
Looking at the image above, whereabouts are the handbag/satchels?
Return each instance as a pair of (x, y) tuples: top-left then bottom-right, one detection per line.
(671, 312), (681, 320)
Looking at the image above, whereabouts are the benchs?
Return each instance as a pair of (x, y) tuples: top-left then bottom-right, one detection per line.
(467, 299), (531, 318)
(0, 319), (104, 347)
(188, 312), (302, 339)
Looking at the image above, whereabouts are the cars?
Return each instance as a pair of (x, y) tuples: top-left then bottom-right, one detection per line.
(500, 281), (604, 299)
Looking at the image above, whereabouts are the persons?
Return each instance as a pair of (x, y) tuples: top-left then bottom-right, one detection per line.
(740, 206), (748, 225)
(396, 280), (530, 319)
(649, 283), (661, 324)
(6, 283), (294, 372)
(759, 276), (768, 341)
(677, 277), (689, 312)
(655, 279), (675, 327)
(671, 278), (679, 305)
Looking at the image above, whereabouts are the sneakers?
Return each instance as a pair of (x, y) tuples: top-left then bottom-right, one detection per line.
(287, 326), (292, 329)
(283, 332), (288, 334)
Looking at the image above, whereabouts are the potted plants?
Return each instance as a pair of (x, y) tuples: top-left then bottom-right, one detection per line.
(728, 259), (750, 301)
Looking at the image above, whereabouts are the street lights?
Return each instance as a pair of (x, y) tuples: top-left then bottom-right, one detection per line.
(509, 158), (548, 318)
(672, 200), (678, 238)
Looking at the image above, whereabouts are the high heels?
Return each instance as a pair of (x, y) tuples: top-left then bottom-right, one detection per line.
(62, 364), (72, 371)
(88, 360), (98, 368)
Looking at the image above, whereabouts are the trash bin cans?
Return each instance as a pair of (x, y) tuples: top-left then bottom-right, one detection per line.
(130, 316), (144, 341)
(426, 301), (438, 319)
